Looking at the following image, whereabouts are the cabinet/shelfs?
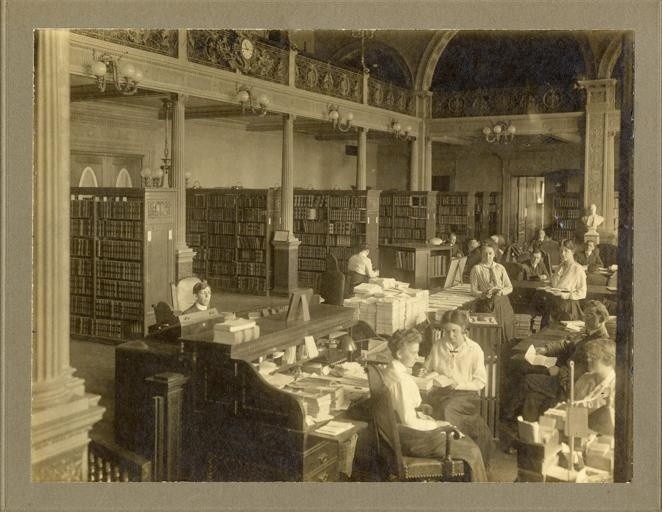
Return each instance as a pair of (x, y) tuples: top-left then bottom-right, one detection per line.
(378, 190), (441, 245)
(185, 187), (279, 298)
(69, 185), (178, 344)
(291, 188), (382, 289)
(547, 190), (582, 243)
(440, 193), (498, 243)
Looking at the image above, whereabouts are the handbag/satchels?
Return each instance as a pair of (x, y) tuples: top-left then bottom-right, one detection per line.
(476, 299), (494, 313)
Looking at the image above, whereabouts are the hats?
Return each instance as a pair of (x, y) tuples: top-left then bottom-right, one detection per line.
(468, 240), (480, 251)
(388, 329), (421, 353)
(193, 282), (208, 294)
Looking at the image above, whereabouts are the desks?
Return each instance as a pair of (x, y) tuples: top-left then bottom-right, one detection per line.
(183, 356), (376, 480)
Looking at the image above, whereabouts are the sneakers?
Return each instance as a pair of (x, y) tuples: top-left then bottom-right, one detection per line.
(501, 408), (513, 420)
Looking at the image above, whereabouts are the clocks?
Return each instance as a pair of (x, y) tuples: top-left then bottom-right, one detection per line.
(240, 37), (255, 62)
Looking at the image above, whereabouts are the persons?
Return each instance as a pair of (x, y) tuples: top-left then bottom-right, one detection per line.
(581, 203), (606, 230)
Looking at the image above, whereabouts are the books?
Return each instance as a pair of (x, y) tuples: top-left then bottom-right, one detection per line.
(551, 192), (581, 242)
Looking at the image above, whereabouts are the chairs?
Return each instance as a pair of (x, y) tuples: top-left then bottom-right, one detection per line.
(168, 275), (212, 320)
(364, 361), (466, 480)
(316, 253), (346, 308)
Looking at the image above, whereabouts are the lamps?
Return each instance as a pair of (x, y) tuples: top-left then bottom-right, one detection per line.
(481, 118), (516, 145)
(236, 82), (270, 118)
(325, 104), (354, 132)
(90, 50), (143, 98)
(139, 100), (191, 187)
(391, 118), (413, 142)
